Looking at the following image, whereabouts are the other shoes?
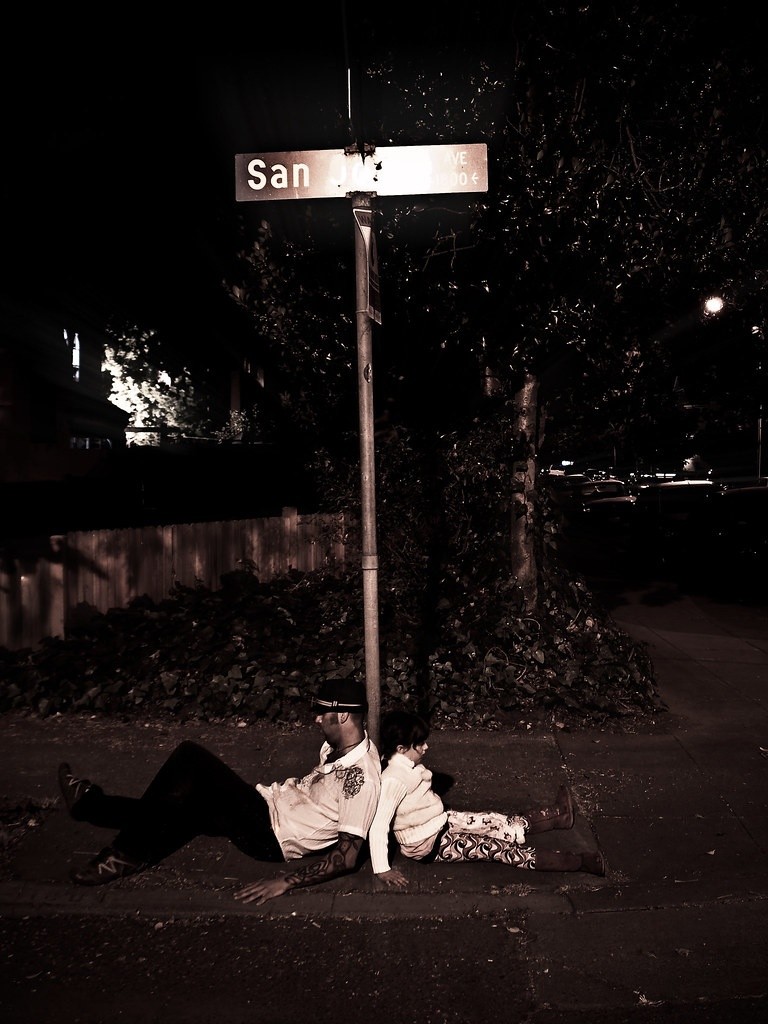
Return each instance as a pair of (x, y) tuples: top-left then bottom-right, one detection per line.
(70, 846), (145, 884)
(58, 761), (92, 811)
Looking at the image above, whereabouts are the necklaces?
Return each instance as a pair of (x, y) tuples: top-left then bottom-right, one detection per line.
(339, 741), (360, 752)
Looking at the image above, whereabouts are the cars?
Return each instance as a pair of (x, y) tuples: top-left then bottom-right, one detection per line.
(535, 465), (768, 602)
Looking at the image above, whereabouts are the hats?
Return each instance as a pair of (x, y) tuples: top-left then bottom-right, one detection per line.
(309, 679), (367, 713)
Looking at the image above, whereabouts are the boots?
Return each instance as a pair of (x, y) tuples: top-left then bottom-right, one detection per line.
(521, 784), (574, 834)
(534, 847), (605, 877)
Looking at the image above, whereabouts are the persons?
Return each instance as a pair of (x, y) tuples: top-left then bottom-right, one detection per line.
(55, 679), (380, 905)
(368, 717), (604, 886)
